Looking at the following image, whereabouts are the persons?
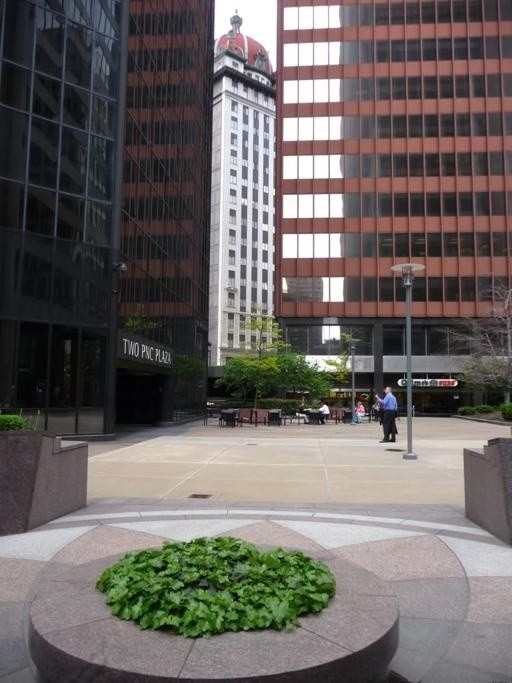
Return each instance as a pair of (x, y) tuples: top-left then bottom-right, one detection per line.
(294, 401), (311, 424)
(374, 385), (399, 442)
(355, 400), (366, 424)
(373, 399), (381, 422)
(318, 401), (331, 424)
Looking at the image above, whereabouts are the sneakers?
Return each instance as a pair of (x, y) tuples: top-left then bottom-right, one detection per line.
(380, 440), (395, 443)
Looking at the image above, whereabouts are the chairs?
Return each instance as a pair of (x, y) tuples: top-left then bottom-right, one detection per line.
(218, 402), (341, 428)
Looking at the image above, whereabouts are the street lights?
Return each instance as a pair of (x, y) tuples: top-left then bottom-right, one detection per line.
(389, 261), (429, 460)
(346, 334), (363, 427)
